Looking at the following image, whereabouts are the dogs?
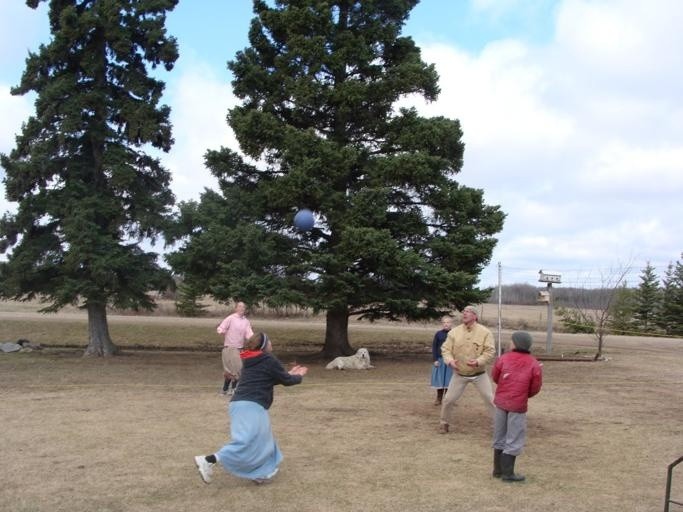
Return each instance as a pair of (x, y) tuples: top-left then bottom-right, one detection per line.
(324, 348), (375, 371)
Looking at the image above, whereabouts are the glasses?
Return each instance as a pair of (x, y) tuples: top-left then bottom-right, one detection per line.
(461, 309), (472, 314)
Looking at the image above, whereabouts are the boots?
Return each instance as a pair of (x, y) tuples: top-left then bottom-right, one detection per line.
(491, 448), (503, 477)
(433, 389), (444, 407)
(499, 451), (526, 483)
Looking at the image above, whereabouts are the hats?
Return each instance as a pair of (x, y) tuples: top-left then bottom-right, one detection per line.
(512, 331), (533, 351)
(465, 305), (479, 322)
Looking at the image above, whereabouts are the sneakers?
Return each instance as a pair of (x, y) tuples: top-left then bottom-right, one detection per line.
(195, 454), (216, 484)
(439, 423), (449, 434)
(229, 388), (235, 394)
(256, 476), (274, 489)
(220, 389), (226, 394)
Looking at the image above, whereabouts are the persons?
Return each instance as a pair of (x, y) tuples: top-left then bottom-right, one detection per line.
(431, 315), (452, 405)
(195, 333), (308, 485)
(217, 301), (254, 396)
(439, 305), (496, 434)
(492, 332), (542, 481)
